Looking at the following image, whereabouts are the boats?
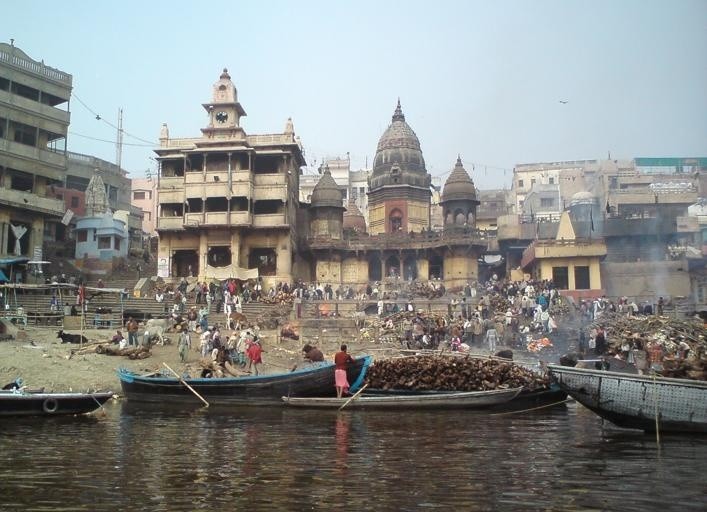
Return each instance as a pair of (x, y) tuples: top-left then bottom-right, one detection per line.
(0, 389), (114, 417)
(119, 353), (373, 404)
(345, 384), (569, 406)
(282, 384), (523, 407)
(541, 362), (707, 434)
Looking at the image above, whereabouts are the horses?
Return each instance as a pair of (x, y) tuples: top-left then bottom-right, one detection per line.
(560, 352), (583, 367)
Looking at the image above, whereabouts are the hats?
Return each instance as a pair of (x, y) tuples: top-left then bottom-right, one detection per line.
(535, 308), (537, 310)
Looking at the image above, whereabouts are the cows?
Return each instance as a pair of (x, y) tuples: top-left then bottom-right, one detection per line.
(142, 325), (164, 346)
(145, 318), (168, 336)
(303, 343), (324, 361)
(280, 329), (299, 342)
(57, 329), (88, 344)
(226, 312), (248, 330)
